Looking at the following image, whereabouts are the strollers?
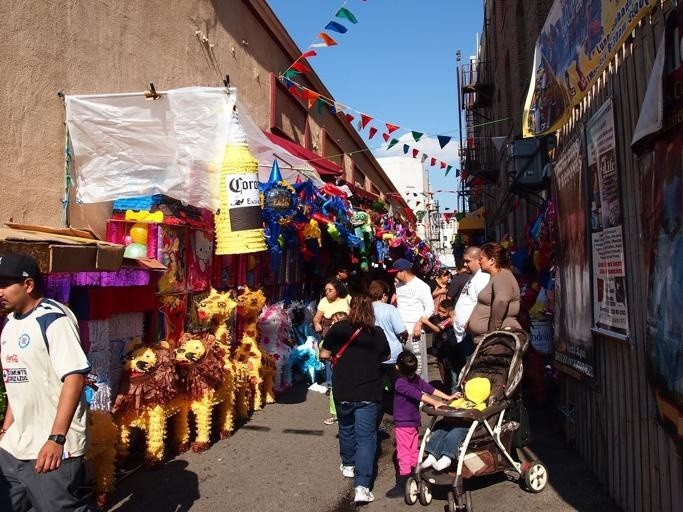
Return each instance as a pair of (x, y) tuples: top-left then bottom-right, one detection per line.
(403, 328), (550, 512)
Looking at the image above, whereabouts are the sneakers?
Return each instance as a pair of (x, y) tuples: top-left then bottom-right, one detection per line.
(354, 485), (374, 502)
(324, 417), (338, 425)
(340, 464), (355, 478)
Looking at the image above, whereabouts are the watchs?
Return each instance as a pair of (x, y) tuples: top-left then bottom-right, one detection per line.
(48, 434), (66, 445)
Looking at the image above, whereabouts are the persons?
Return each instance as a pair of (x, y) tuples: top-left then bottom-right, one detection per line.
(1, 253), (102, 512)
(313, 277), (354, 398)
(469, 240), (523, 464)
(323, 310), (348, 424)
(452, 245), (490, 383)
(416, 376), (494, 476)
(320, 294), (392, 503)
(367, 277), (408, 441)
(384, 351), (463, 498)
(333, 259), (471, 409)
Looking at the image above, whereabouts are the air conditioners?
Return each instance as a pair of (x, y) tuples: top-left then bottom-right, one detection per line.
(502, 138), (543, 190)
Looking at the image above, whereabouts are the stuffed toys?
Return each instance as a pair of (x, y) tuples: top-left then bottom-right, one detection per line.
(81, 282), (325, 508)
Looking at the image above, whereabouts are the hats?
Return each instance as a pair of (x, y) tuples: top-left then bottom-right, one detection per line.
(0, 254), (42, 277)
(388, 258), (413, 272)
